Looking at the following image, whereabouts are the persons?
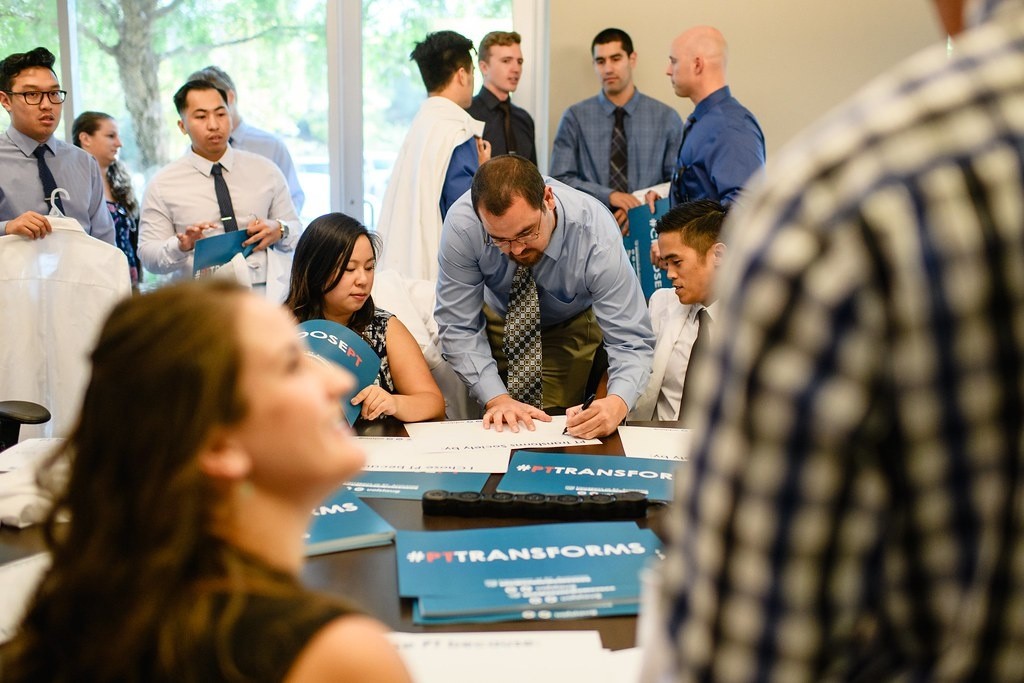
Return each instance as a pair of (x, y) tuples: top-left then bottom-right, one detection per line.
(467, 32), (538, 166)
(627, 200), (732, 422)
(285, 212), (446, 423)
(187, 64), (305, 216)
(645, 25), (766, 270)
(368, 29), (491, 420)
(433, 154), (657, 440)
(634, 0), (1024, 682)
(0, 46), (116, 248)
(0, 280), (414, 683)
(73, 111), (145, 297)
(550, 28), (685, 237)
(135, 82), (303, 292)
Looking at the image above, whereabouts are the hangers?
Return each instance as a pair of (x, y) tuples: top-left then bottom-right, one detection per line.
(48, 187), (71, 218)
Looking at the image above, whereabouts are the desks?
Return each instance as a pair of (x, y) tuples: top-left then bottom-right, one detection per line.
(0, 412), (689, 683)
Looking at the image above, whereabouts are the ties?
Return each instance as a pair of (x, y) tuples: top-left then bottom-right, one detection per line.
(210, 163), (238, 232)
(502, 264), (542, 413)
(33, 144), (65, 216)
(498, 102), (517, 154)
(609, 106), (628, 214)
(678, 310), (712, 420)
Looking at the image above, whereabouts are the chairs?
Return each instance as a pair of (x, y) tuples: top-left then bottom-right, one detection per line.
(374, 271), (469, 419)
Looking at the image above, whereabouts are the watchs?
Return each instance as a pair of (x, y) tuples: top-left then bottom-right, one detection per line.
(277, 220), (289, 241)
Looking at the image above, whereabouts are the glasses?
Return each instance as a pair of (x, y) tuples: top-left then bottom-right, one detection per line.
(484, 202), (543, 249)
(5, 89), (67, 106)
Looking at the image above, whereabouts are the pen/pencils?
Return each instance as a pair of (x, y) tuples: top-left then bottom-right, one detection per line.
(563, 394), (596, 435)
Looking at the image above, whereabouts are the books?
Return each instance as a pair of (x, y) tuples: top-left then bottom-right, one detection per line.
(496, 450), (686, 502)
(300, 488), (395, 559)
(341, 471), (490, 500)
(394, 520), (670, 626)
